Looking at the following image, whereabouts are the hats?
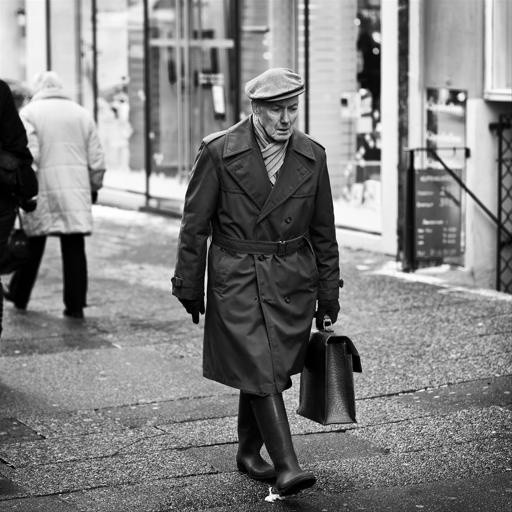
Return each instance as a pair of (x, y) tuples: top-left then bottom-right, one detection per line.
(244, 68), (306, 102)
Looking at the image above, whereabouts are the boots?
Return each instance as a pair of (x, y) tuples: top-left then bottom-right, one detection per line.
(242, 388), (317, 497)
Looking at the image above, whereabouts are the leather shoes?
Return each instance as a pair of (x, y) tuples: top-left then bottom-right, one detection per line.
(0, 280), (27, 310)
(62, 305), (84, 319)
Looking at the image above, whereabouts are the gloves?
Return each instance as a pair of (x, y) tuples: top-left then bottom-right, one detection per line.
(313, 299), (341, 332)
(20, 199), (37, 212)
(176, 294), (206, 324)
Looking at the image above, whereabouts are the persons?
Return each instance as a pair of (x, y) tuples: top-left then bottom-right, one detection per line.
(1, 70), (106, 334)
(171, 67), (344, 496)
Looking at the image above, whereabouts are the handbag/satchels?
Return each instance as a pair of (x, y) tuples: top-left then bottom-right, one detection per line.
(2, 206), (31, 275)
(295, 315), (363, 426)
